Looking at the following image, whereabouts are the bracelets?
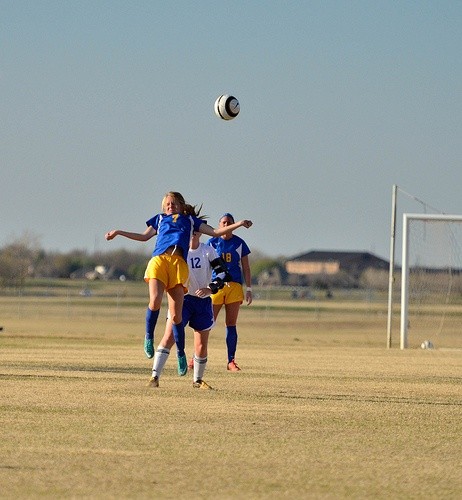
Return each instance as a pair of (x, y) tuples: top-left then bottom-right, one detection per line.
(246, 287), (252, 291)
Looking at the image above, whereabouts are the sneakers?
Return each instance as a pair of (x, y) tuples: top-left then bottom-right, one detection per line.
(144, 334), (154, 359)
(146, 377), (159, 388)
(177, 353), (187, 376)
(227, 360), (241, 372)
(192, 380), (213, 390)
(187, 357), (194, 373)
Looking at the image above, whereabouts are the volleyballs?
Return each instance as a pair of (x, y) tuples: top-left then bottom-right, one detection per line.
(214, 94), (241, 121)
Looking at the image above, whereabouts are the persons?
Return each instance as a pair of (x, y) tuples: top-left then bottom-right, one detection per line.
(104, 191), (253, 376)
(146, 216), (230, 390)
(188, 212), (253, 371)
